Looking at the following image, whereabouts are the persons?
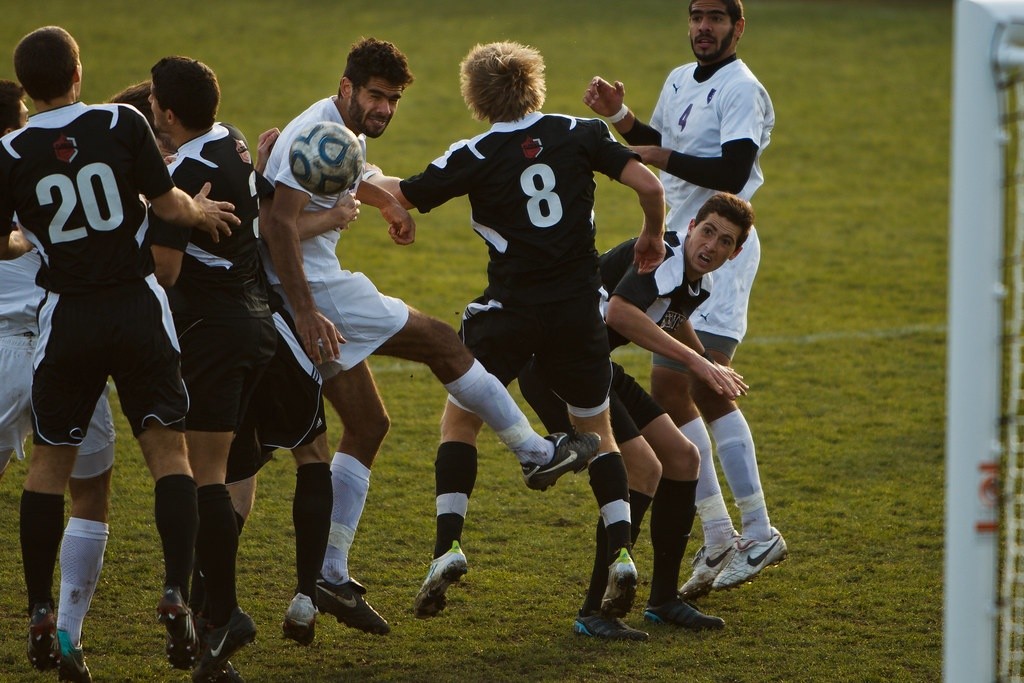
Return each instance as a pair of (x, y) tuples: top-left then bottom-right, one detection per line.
(257, 39), (602, 633)
(354, 40), (667, 619)
(0, 26), (360, 683)
(516, 191), (755, 640)
(584, 0), (789, 598)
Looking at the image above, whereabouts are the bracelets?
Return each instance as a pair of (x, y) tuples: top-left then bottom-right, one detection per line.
(606, 104), (629, 124)
(363, 165), (381, 182)
(702, 350), (716, 366)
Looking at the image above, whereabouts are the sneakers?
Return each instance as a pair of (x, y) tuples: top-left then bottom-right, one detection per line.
(643, 593), (726, 630)
(678, 530), (742, 598)
(518, 430), (601, 493)
(711, 525), (789, 590)
(193, 612), (246, 683)
(191, 608), (258, 683)
(295, 572), (391, 635)
(56, 628), (94, 682)
(413, 539), (468, 620)
(573, 607), (650, 640)
(601, 545), (638, 618)
(282, 592), (319, 644)
(156, 584), (200, 670)
(26, 599), (61, 672)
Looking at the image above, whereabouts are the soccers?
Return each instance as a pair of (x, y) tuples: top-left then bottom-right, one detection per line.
(286, 120), (365, 197)
